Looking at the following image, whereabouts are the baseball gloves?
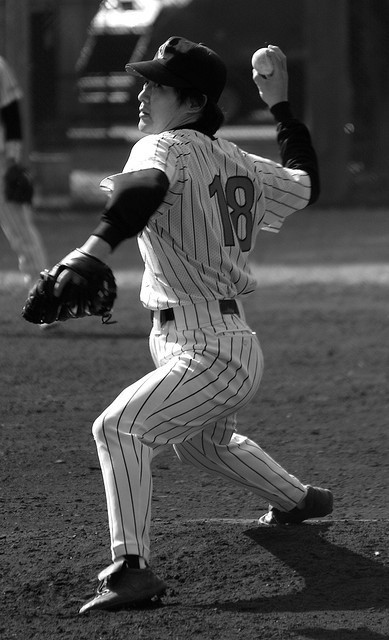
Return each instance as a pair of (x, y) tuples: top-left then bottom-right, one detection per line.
(20, 246), (116, 325)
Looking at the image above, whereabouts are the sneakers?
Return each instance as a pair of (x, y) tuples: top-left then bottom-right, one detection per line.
(258, 485), (334, 527)
(78, 559), (169, 614)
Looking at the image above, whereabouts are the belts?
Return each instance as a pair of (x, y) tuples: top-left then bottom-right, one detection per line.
(151, 298), (238, 323)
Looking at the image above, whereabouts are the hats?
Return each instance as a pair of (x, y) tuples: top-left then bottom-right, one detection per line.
(125, 37), (227, 102)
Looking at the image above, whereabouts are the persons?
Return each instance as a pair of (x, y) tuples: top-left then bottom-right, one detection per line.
(20, 35), (334, 617)
(0, 55), (48, 288)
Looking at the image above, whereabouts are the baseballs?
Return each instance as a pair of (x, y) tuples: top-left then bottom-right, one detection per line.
(252, 48), (274, 75)
